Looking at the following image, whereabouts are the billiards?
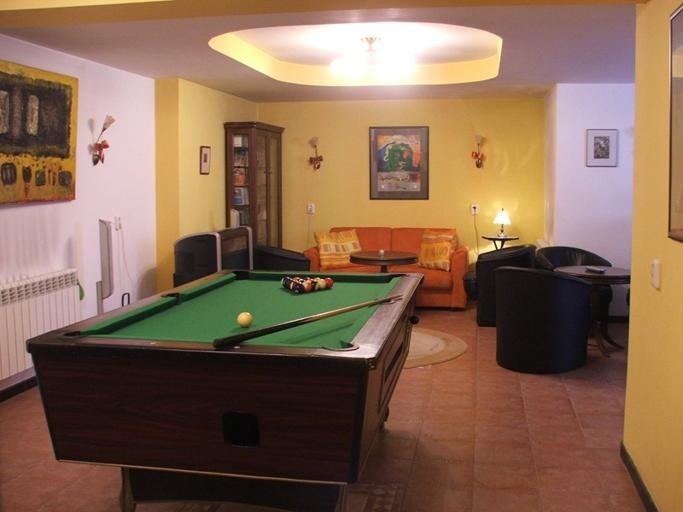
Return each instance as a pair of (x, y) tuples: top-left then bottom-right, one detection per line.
(281, 277), (334, 294)
(237, 312), (253, 327)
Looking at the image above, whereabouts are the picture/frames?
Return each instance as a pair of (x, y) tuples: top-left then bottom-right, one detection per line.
(669, 2), (683, 244)
(368, 125), (428, 200)
(199, 146), (210, 175)
(585, 128), (618, 167)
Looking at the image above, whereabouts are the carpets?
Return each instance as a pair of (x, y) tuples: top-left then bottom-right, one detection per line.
(400, 328), (467, 369)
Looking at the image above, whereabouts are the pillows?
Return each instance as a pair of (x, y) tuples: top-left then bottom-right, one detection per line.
(417, 229), (455, 271)
(313, 228), (362, 269)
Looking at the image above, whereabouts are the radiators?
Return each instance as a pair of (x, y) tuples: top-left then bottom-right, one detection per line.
(1, 270), (77, 381)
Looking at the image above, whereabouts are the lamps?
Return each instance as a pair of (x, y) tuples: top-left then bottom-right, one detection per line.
(491, 208), (511, 237)
(307, 136), (323, 170)
(470, 135), (483, 168)
(329, 31), (412, 81)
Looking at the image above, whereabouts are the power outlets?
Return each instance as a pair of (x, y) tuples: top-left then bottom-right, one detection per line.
(306, 203), (314, 215)
(647, 261), (658, 290)
(470, 204), (478, 215)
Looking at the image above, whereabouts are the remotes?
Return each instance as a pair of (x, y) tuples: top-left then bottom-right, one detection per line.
(586, 266), (606, 272)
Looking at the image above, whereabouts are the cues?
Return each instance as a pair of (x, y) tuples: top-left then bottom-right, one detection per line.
(213, 294), (405, 349)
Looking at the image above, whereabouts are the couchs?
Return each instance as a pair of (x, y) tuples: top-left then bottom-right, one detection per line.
(173, 229), (310, 289)
(304, 226), (468, 308)
(496, 266), (592, 375)
(536, 246), (612, 302)
(474, 243), (536, 327)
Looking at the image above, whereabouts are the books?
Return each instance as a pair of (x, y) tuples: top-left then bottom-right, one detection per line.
(229, 208), (247, 228)
(233, 135), (247, 147)
(233, 150), (248, 167)
(232, 187), (250, 206)
(232, 167), (249, 186)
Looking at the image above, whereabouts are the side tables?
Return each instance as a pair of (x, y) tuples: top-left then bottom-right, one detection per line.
(480, 235), (518, 249)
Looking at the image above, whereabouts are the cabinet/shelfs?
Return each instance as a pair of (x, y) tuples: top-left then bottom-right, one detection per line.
(223, 121), (284, 249)
